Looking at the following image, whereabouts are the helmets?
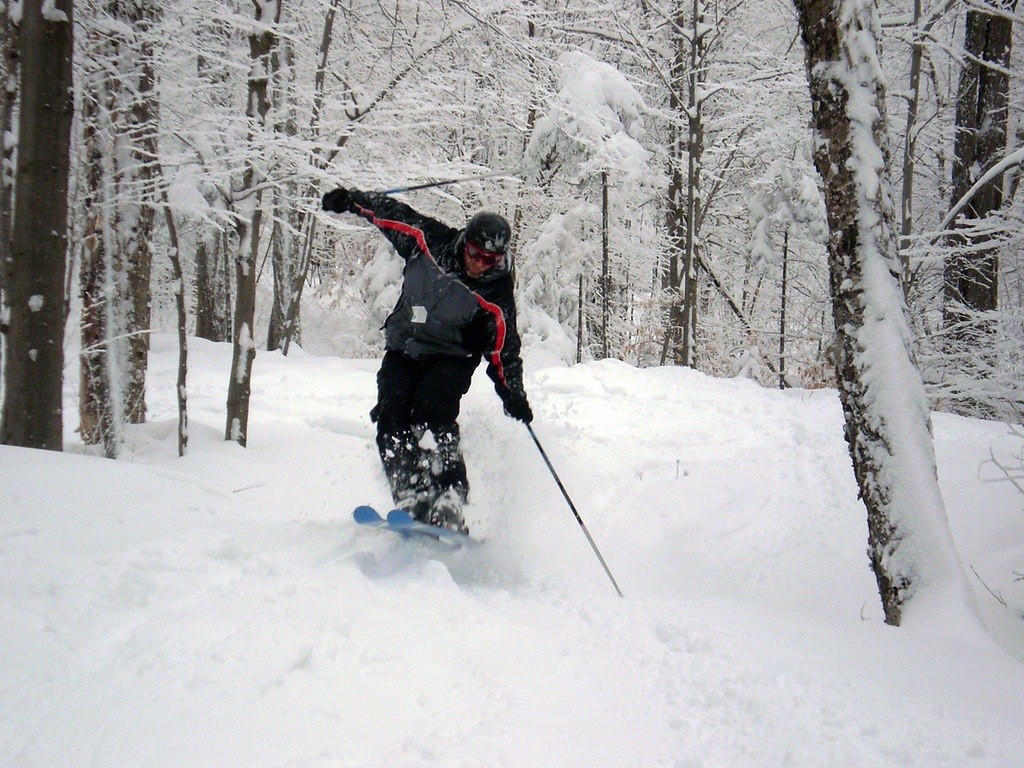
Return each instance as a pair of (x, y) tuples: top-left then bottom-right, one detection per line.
(464, 211), (513, 256)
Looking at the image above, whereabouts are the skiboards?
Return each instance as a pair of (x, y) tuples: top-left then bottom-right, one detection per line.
(352, 506), (482, 553)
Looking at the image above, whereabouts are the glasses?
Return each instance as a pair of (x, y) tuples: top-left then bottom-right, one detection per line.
(463, 239), (506, 267)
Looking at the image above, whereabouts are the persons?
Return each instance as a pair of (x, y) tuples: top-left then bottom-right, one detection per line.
(322, 187), (533, 533)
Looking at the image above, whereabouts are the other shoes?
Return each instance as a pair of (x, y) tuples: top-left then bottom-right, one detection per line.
(395, 490), (430, 521)
(430, 485), (465, 532)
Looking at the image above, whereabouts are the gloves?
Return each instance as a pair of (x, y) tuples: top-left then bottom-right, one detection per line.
(322, 189), (353, 214)
(504, 392), (534, 425)
